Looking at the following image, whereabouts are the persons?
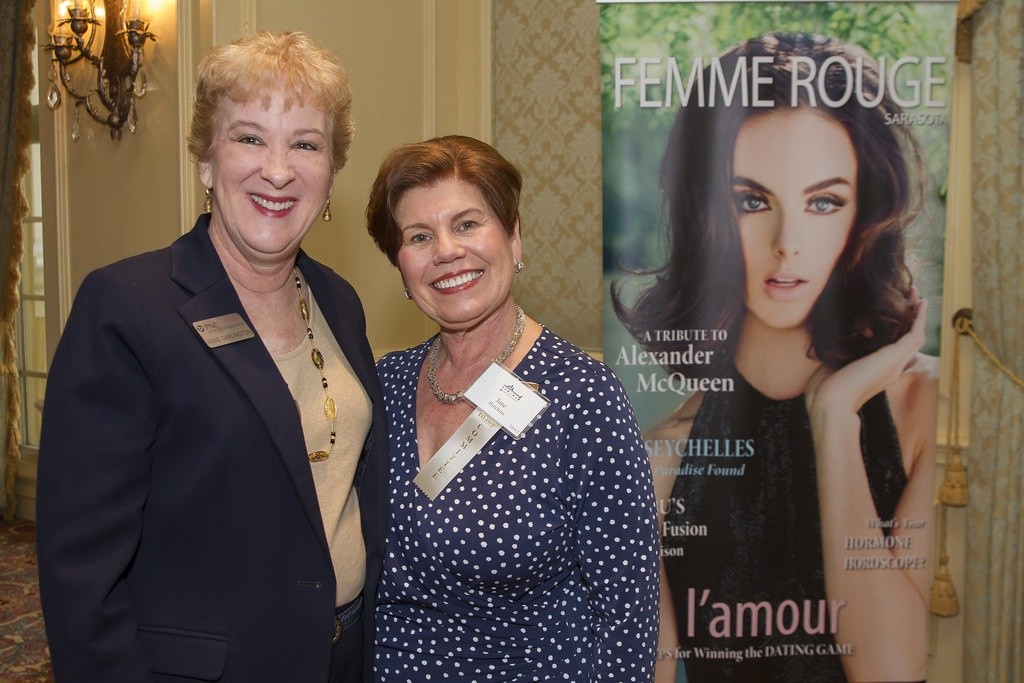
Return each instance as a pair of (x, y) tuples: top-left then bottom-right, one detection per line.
(362, 132), (659, 683)
(36, 32), (388, 683)
(611, 30), (938, 683)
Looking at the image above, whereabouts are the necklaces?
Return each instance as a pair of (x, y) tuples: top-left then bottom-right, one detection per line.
(428, 304), (525, 404)
(287, 267), (337, 463)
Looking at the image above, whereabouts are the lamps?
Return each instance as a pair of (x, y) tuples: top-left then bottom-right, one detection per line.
(43, 0), (156, 148)
